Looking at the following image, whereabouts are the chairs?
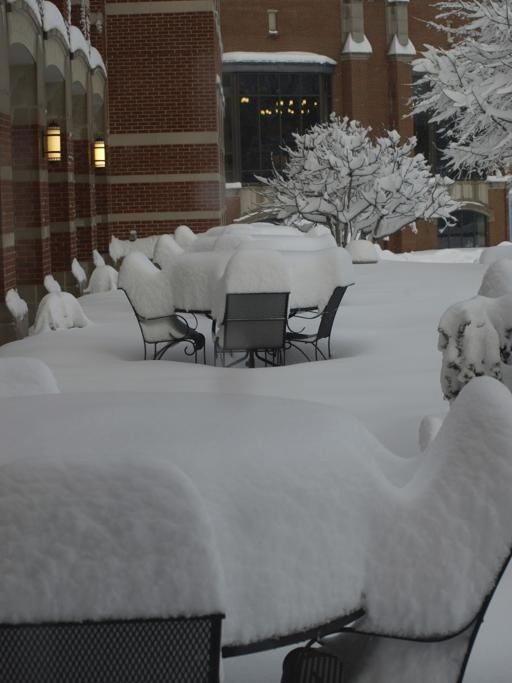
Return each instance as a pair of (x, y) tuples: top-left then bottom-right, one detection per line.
(262, 277), (357, 368)
(0, 451), (234, 683)
(269, 373), (512, 683)
(113, 277), (208, 365)
(209, 287), (291, 369)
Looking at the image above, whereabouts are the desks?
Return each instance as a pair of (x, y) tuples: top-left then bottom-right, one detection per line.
(219, 595), (370, 662)
(168, 302), (320, 370)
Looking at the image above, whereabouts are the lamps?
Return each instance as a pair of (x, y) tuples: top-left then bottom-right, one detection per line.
(40, 118), (64, 164)
(88, 133), (108, 171)
